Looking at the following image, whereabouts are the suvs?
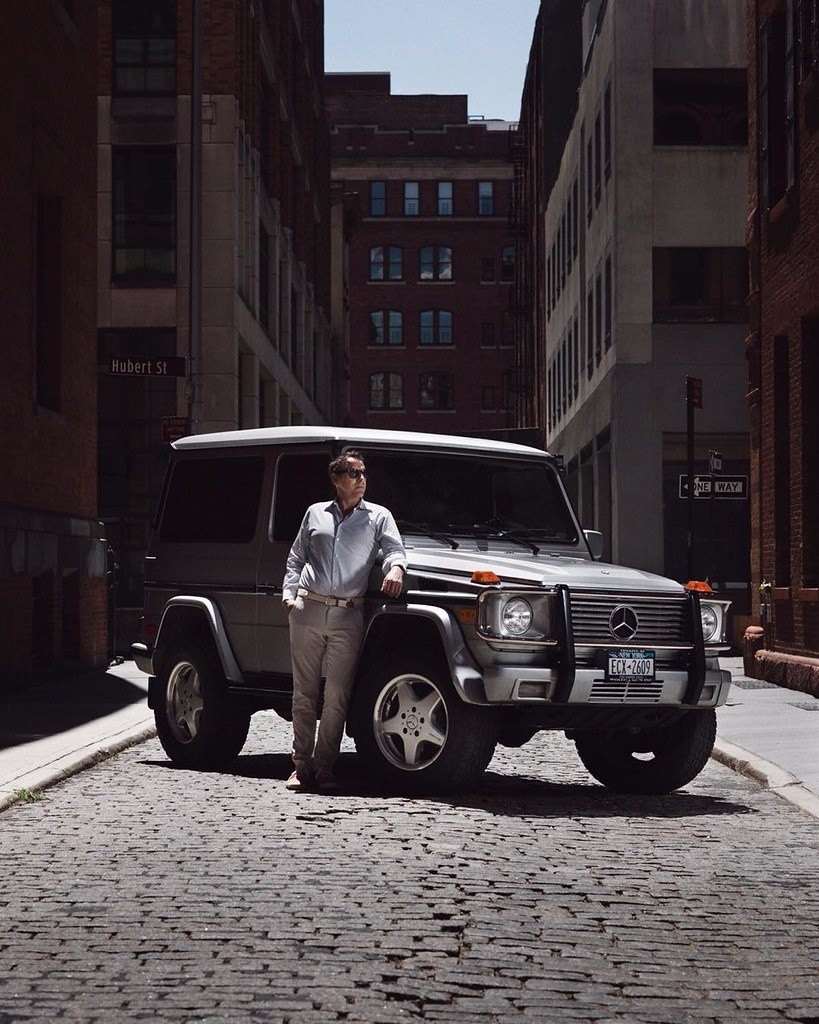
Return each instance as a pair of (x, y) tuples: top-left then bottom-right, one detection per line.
(131, 425), (733, 795)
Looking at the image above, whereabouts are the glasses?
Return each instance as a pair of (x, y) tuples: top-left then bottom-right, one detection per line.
(335, 469), (369, 479)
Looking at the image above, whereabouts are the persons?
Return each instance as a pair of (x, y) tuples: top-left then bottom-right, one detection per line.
(283, 453), (408, 789)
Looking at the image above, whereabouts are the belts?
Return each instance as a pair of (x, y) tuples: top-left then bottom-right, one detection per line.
(296, 588), (365, 609)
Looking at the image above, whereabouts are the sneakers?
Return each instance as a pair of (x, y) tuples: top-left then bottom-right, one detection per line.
(314, 763), (347, 790)
(285, 765), (308, 790)
